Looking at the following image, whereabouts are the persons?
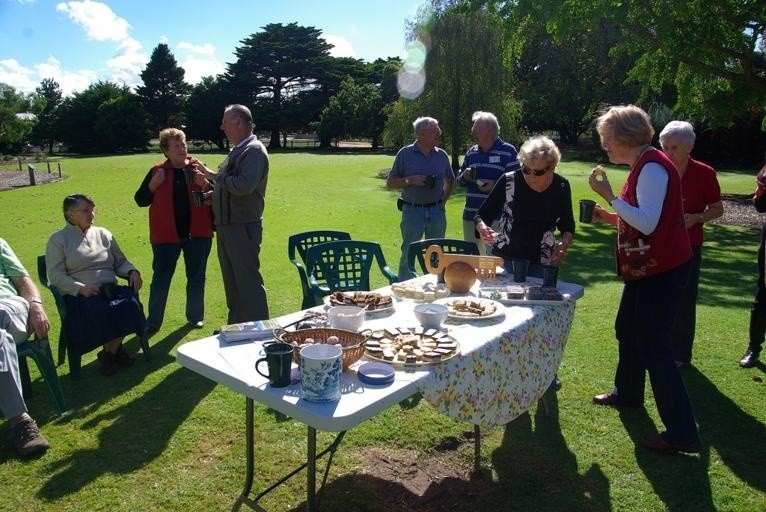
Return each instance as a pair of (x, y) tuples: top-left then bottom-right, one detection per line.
(659, 120), (724, 369)
(193, 105), (269, 326)
(135, 127), (215, 336)
(474, 134), (575, 390)
(387, 117), (456, 282)
(45, 195), (144, 376)
(587, 105), (701, 453)
(0, 238), (50, 457)
(740, 165), (766, 367)
(457, 112), (522, 254)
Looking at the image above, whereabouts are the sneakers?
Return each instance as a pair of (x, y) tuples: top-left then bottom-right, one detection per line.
(97, 349), (116, 376)
(740, 350), (760, 367)
(115, 343), (136, 367)
(9, 417), (50, 454)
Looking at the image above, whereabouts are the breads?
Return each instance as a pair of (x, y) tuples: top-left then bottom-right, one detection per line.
(288, 280), (563, 364)
(594, 165), (605, 176)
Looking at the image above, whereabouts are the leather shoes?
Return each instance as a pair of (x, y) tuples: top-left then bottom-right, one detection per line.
(593, 394), (643, 407)
(640, 433), (697, 453)
(187, 320), (203, 327)
(143, 323), (159, 338)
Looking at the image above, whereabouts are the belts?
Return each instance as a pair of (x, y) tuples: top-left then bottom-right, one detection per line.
(402, 200), (443, 208)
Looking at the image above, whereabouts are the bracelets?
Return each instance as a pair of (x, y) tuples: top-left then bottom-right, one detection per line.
(609, 196), (618, 207)
(700, 214), (703, 223)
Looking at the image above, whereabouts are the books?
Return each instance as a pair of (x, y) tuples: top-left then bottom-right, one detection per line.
(220, 319), (282, 343)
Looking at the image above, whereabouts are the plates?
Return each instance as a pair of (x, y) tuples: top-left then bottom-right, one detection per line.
(364, 328), (462, 367)
(390, 287), (452, 304)
(432, 297), (506, 320)
(322, 291), (397, 313)
(476, 265), (507, 275)
(479, 286), (566, 306)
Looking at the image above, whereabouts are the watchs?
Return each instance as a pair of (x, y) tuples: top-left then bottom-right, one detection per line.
(404, 177), (410, 186)
(28, 298), (42, 304)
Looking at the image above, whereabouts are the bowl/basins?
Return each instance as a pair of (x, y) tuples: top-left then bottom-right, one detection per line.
(414, 303), (449, 329)
(327, 306), (365, 334)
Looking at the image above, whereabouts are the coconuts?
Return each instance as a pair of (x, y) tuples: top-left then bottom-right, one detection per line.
(444, 261), (477, 293)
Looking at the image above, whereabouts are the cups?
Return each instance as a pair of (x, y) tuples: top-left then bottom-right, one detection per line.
(579, 199), (597, 224)
(255, 343), (294, 387)
(192, 190), (204, 208)
(542, 265), (559, 288)
(511, 258), (530, 282)
(183, 168), (195, 184)
(425, 175), (437, 188)
(470, 166), (480, 182)
(99, 282), (117, 301)
(299, 342), (341, 403)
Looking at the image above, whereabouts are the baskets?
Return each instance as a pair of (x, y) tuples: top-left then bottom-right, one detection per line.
(272, 326), (373, 370)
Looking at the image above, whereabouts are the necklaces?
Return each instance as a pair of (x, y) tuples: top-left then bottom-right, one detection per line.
(630, 144), (650, 171)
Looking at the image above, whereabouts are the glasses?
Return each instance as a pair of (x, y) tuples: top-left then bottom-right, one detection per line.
(521, 161), (552, 176)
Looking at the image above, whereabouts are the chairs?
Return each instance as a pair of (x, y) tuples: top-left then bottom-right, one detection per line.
(18, 324), (70, 418)
(285, 229), (481, 308)
(34, 251), (151, 381)
